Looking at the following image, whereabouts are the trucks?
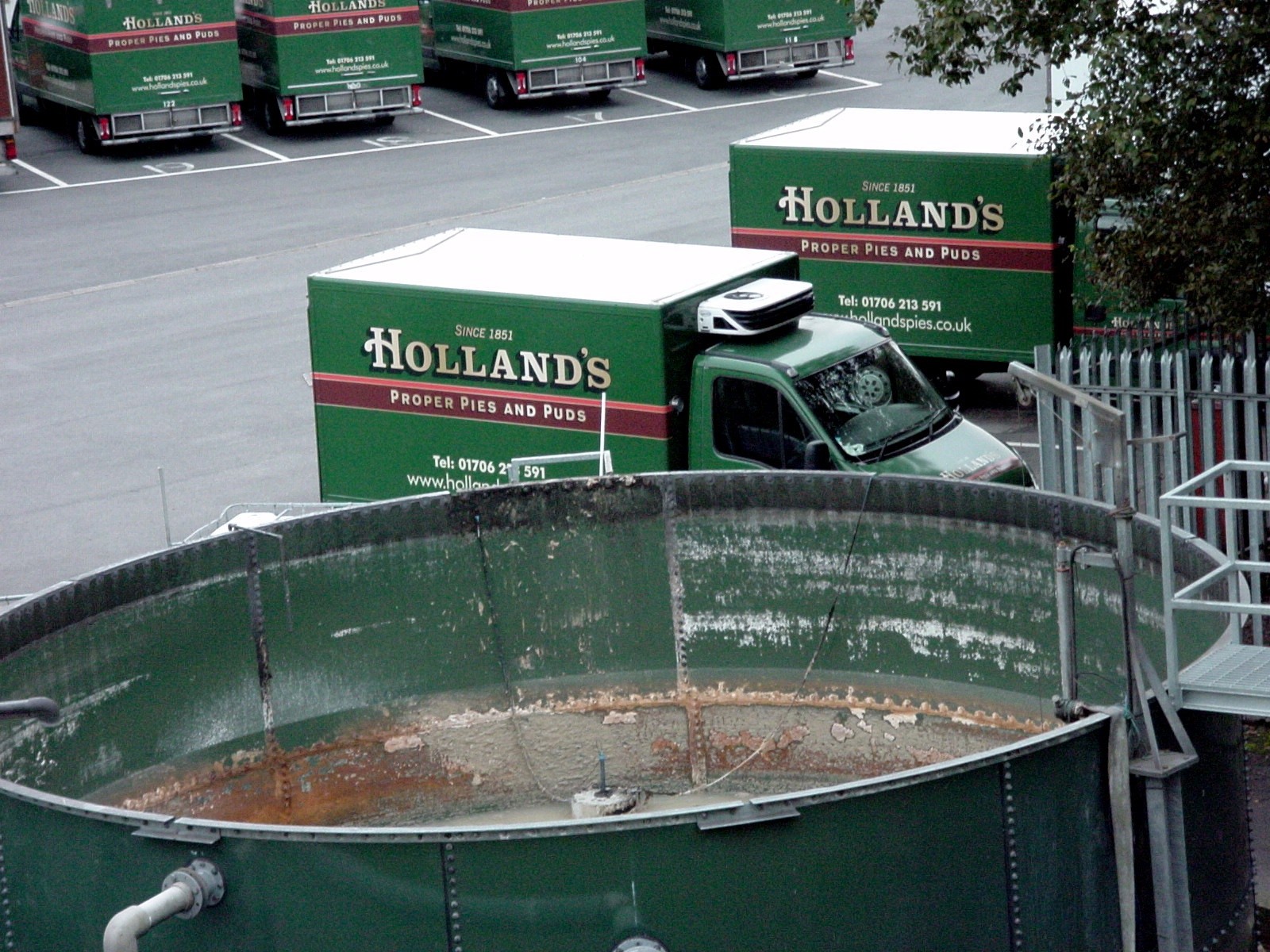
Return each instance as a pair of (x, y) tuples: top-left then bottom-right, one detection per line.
(417, 0), (648, 110)
(305, 225), (1037, 518)
(640, 0), (858, 91)
(227, 0), (425, 137)
(7, 0), (246, 156)
(0, 0), (23, 160)
(727, 104), (1234, 415)
